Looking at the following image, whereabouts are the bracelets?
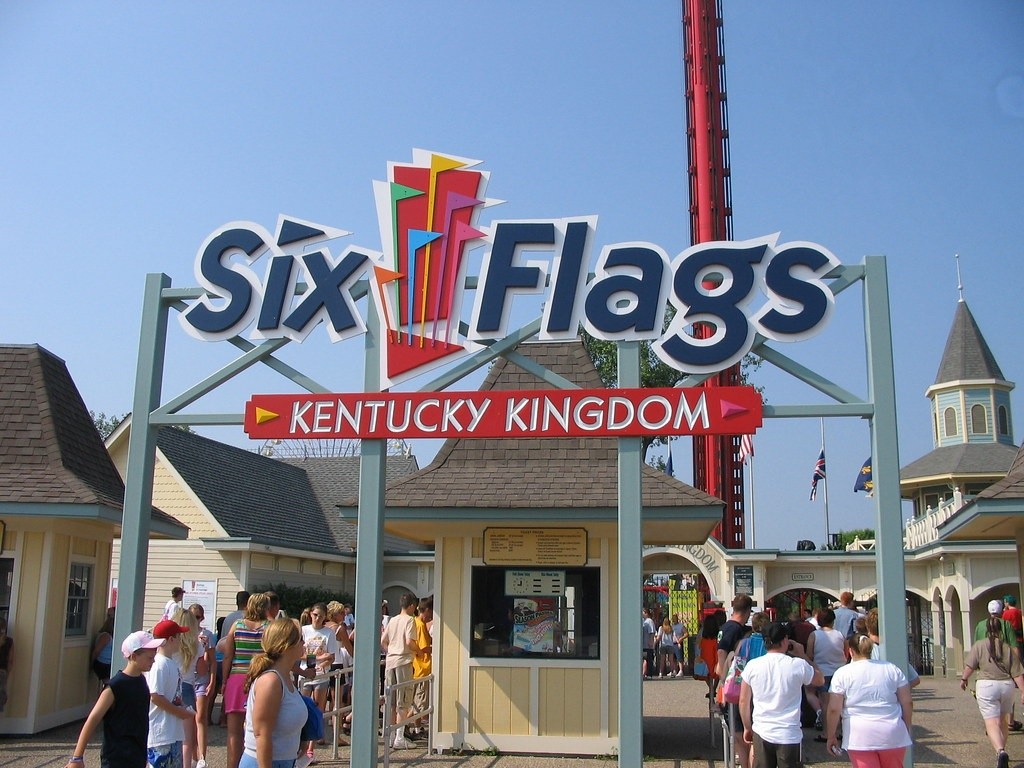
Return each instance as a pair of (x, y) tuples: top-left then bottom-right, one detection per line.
(962, 679), (967, 681)
(70, 756), (83, 762)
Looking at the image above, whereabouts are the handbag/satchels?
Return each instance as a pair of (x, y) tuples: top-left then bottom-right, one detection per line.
(295, 694), (324, 740)
(724, 638), (748, 704)
(693, 657), (709, 681)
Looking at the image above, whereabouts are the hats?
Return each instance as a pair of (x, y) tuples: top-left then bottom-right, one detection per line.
(153, 620), (190, 638)
(121, 631), (166, 657)
(761, 621), (789, 644)
(172, 587), (185, 596)
(988, 600), (1002, 614)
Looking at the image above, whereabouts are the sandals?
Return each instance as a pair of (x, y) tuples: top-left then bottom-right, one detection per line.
(1007, 720), (1023, 731)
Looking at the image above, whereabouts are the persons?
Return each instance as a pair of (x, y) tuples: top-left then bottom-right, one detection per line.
(1002, 596), (1024, 666)
(960, 617), (1024, 768)
(827, 633), (913, 768)
(716, 592), (920, 768)
(739, 622), (824, 768)
(239, 618), (310, 768)
(643, 608), (689, 678)
(974, 600), (1024, 730)
(91, 607), (116, 682)
(64, 631), (166, 768)
(0, 616), (13, 711)
(164, 586), (433, 768)
(146, 620), (196, 768)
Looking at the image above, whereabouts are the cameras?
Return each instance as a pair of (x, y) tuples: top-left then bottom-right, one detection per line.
(831, 745), (842, 756)
(788, 643), (793, 651)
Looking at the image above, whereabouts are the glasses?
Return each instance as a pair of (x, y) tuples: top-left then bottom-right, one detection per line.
(310, 612), (325, 621)
(194, 615), (203, 620)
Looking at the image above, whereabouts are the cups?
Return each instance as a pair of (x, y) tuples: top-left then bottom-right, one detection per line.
(788, 641), (794, 651)
(307, 654), (316, 669)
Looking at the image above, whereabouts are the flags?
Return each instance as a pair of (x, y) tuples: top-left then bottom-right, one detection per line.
(854, 457), (873, 492)
(665, 453), (672, 476)
(740, 435), (754, 465)
(810, 450), (826, 501)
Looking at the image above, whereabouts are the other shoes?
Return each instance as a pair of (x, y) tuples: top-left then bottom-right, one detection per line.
(676, 671), (683, 676)
(195, 754), (208, 768)
(995, 749), (1009, 768)
(706, 692), (716, 698)
(210, 700), (429, 764)
(191, 759), (197, 768)
(671, 671), (677, 678)
(658, 673), (663, 679)
(799, 710), (844, 744)
(668, 672), (673, 677)
